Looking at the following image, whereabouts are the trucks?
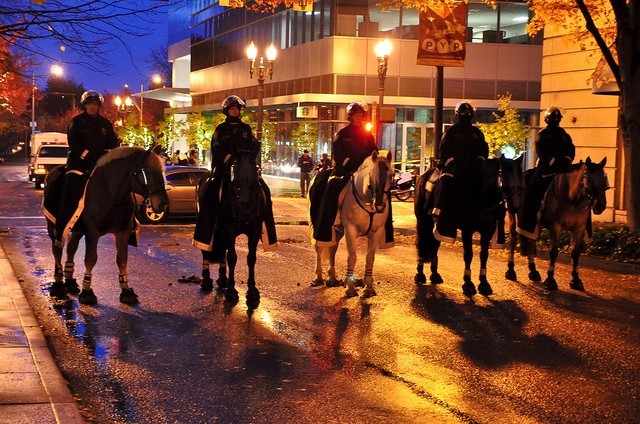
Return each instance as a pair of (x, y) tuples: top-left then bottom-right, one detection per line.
(28, 131), (68, 181)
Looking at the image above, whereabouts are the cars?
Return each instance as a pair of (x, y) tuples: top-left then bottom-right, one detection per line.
(131, 167), (208, 223)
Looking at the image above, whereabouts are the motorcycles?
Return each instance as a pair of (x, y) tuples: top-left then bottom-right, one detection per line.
(390, 172), (416, 200)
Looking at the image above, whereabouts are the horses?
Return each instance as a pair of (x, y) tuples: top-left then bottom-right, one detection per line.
(412, 152), (525, 295)
(504, 154), (610, 291)
(194, 139), (267, 299)
(306, 149), (395, 298)
(44, 141), (169, 305)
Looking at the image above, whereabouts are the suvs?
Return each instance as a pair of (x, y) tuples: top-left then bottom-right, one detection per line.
(31, 144), (70, 188)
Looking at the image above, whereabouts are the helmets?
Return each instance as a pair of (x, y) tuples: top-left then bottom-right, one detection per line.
(222, 95), (246, 112)
(346, 102), (368, 118)
(545, 106), (563, 123)
(79, 90), (104, 109)
(454, 101), (474, 118)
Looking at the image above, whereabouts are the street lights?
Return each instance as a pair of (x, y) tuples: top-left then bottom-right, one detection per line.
(114, 95), (132, 146)
(32, 83), (39, 133)
(246, 40), (277, 176)
(375, 39), (393, 146)
(124, 81), (143, 126)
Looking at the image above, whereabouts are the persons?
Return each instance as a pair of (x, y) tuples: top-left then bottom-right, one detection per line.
(210, 94), (253, 170)
(317, 154), (332, 171)
(170, 149), (180, 165)
(188, 148), (198, 163)
(440, 102), (489, 165)
(331, 104), (377, 177)
(296, 148), (313, 196)
(58, 90), (120, 194)
(533, 108), (575, 168)
